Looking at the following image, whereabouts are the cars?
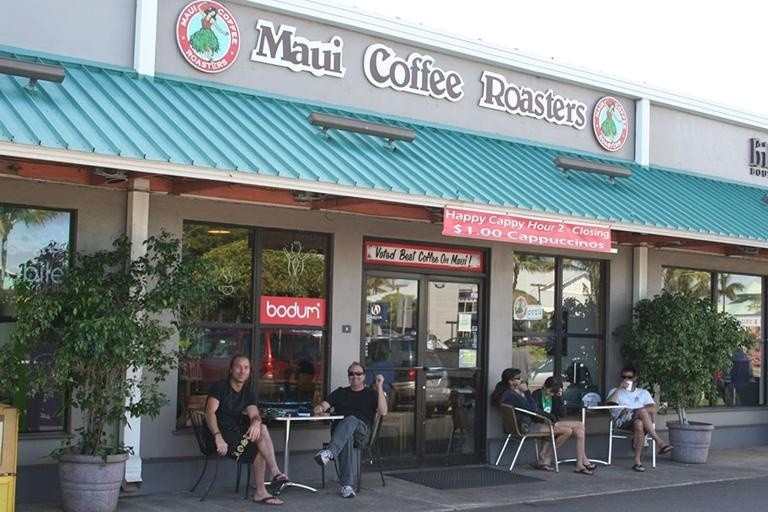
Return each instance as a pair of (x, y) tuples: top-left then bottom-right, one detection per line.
(361, 335), (450, 410)
(529, 358), (573, 388)
(439, 337), (481, 382)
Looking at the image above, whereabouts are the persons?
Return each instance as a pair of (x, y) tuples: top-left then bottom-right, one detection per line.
(284, 359), (314, 400)
(605, 367), (673, 472)
(723, 346), (754, 406)
(313, 362), (388, 498)
(491, 368), (573, 471)
(364, 340), (395, 463)
(512, 339), (531, 389)
(532, 376), (597, 475)
(204, 353), (289, 505)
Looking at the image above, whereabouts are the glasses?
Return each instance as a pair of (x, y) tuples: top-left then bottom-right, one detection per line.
(514, 377), (521, 380)
(621, 376), (634, 379)
(347, 372), (363, 376)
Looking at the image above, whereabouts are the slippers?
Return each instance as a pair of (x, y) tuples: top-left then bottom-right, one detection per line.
(529, 445), (673, 474)
(252, 494), (284, 505)
(271, 473), (290, 486)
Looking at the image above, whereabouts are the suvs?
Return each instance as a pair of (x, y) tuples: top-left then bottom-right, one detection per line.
(183, 330), (323, 401)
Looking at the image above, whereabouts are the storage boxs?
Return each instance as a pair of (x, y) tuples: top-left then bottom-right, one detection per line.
(0, 405), (18, 473)
(0, 473), (16, 512)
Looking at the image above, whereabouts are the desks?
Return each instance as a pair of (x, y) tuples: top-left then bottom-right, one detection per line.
(247, 412), (344, 496)
(551, 402), (629, 467)
(379, 412), (413, 452)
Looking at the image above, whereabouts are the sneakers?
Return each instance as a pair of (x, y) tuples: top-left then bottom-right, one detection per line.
(340, 485), (356, 499)
(314, 449), (334, 469)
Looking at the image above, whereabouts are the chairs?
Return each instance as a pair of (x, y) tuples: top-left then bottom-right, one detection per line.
(607, 399), (656, 468)
(176, 394), (208, 428)
(319, 385), (390, 494)
(494, 403), (562, 473)
(189, 407), (251, 503)
(424, 409), (454, 457)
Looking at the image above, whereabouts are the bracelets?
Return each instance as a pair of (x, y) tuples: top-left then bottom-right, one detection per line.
(212, 432), (222, 437)
(318, 403), (325, 411)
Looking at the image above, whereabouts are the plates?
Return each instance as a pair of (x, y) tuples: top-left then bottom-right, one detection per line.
(512, 296), (528, 320)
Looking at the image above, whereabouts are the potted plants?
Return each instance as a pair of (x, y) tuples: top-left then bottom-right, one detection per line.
(0, 225), (223, 512)
(610, 286), (764, 464)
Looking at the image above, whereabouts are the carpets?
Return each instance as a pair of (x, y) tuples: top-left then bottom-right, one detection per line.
(384, 465), (546, 490)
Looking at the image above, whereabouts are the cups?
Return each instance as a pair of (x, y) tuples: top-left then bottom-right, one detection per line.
(626, 380), (633, 391)
